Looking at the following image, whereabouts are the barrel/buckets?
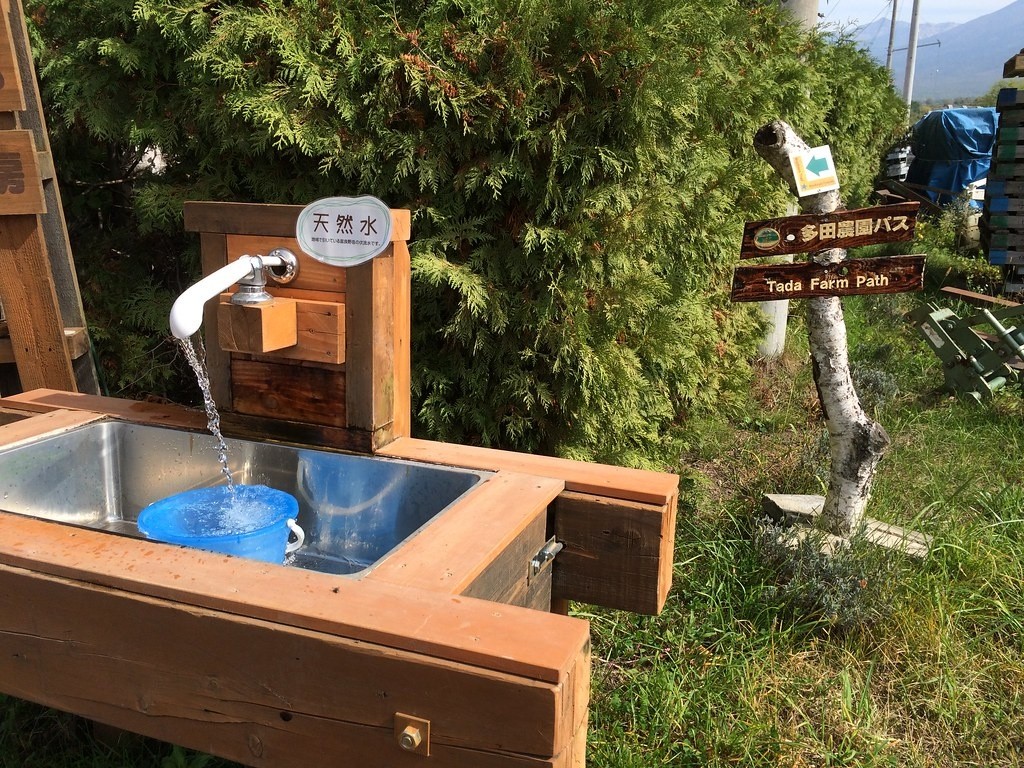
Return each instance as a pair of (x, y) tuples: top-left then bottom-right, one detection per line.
(139, 484), (305, 568)
(297, 452), (407, 558)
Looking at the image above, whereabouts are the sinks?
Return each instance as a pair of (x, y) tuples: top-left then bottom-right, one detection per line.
(0, 416), (499, 582)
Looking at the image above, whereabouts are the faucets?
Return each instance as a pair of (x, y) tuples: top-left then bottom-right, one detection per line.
(168, 253), (288, 341)
